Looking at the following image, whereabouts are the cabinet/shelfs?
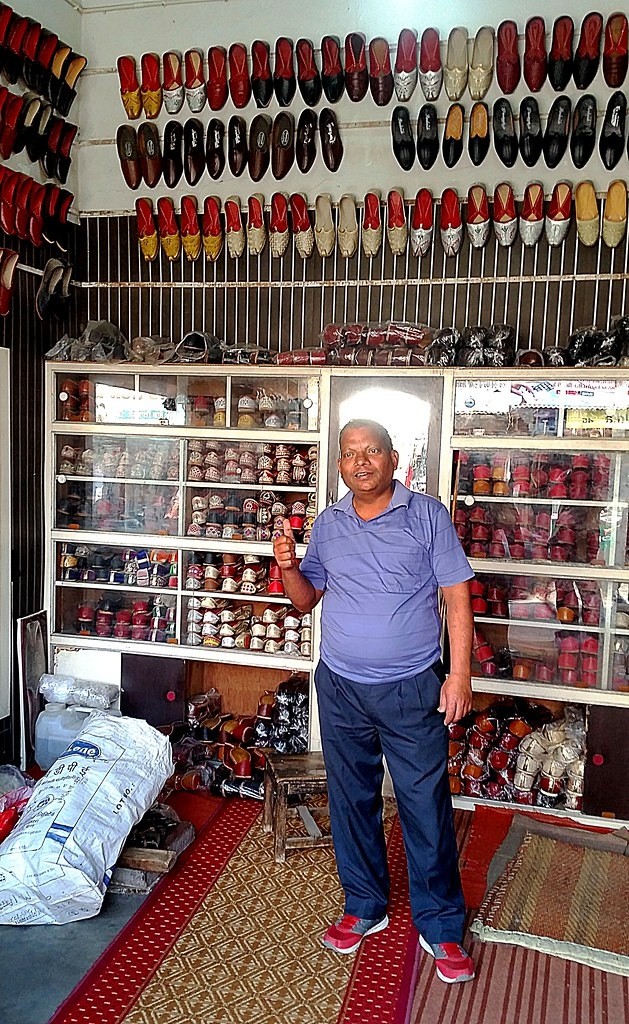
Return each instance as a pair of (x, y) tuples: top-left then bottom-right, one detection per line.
(442, 368), (629, 830)
(36, 358), (322, 745)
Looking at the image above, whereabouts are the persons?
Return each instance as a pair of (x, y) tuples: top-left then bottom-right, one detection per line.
(274, 418), (475, 984)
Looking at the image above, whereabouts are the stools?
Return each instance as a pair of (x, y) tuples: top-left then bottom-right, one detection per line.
(261, 750), (333, 863)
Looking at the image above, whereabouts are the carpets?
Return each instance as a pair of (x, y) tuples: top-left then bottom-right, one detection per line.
(33, 786), (629, 1024)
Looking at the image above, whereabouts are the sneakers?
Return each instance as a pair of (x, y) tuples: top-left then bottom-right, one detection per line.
(419, 933), (475, 984)
(322, 911), (389, 954)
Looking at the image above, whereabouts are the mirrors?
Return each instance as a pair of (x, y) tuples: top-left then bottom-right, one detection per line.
(316, 366), (450, 703)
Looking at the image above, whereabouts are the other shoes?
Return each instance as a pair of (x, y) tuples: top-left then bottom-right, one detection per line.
(54, 259), (74, 320)
(0, 3), (13, 72)
(5, 14), (28, 84)
(0, 93), (23, 159)
(41, 118), (67, 178)
(57, 52), (88, 117)
(56, 382), (316, 658)
(57, 123), (78, 184)
(36, 259), (64, 321)
(13, 92), (41, 153)
(448, 450), (629, 691)
(29, 101), (53, 163)
(0, 164), (74, 253)
(0, 86), (10, 132)
(45, 316), (629, 369)
(0, 248), (19, 317)
(49, 42), (72, 105)
(35, 28), (59, 95)
(162, 682), (309, 800)
(448, 706), (584, 813)
(22, 17), (42, 90)
(116, 9), (629, 263)
